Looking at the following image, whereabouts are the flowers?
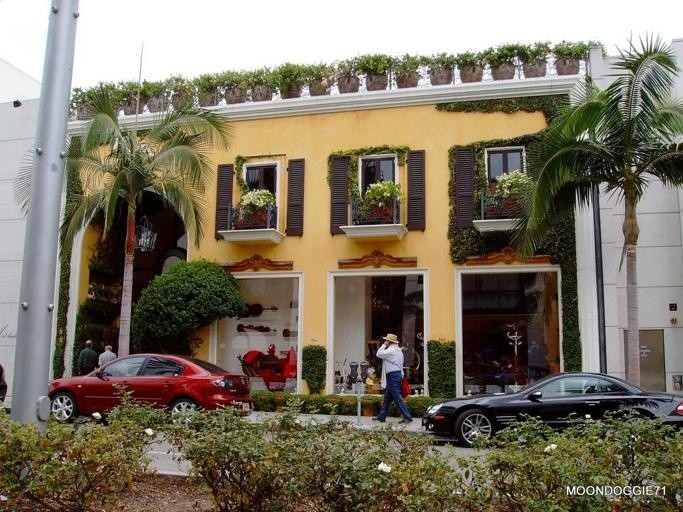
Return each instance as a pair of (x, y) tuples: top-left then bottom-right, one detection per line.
(241, 188), (275, 214)
(494, 168), (531, 198)
(364, 180), (402, 209)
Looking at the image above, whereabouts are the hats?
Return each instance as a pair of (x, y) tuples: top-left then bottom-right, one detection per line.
(383, 333), (400, 344)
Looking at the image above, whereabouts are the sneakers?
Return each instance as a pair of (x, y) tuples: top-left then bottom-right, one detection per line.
(398, 418), (409, 425)
(372, 417), (385, 422)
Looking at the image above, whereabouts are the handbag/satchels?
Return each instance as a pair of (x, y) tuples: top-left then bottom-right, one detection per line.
(401, 378), (412, 398)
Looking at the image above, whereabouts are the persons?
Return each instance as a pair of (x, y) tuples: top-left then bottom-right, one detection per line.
(371, 332), (413, 425)
(98, 345), (117, 368)
(77, 340), (99, 375)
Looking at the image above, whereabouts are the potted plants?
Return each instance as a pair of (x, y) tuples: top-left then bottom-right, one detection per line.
(68, 39), (588, 119)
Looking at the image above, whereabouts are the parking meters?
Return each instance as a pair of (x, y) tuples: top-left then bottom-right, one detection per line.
(348, 361), (370, 425)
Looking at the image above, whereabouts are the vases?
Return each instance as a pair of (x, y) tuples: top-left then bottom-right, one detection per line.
(370, 205), (393, 221)
(233, 208), (267, 228)
(485, 197), (518, 215)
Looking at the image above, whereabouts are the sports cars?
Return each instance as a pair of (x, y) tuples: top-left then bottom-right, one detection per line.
(422, 372), (683, 447)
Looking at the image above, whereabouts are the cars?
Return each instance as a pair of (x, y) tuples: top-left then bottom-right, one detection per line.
(48, 354), (256, 426)
